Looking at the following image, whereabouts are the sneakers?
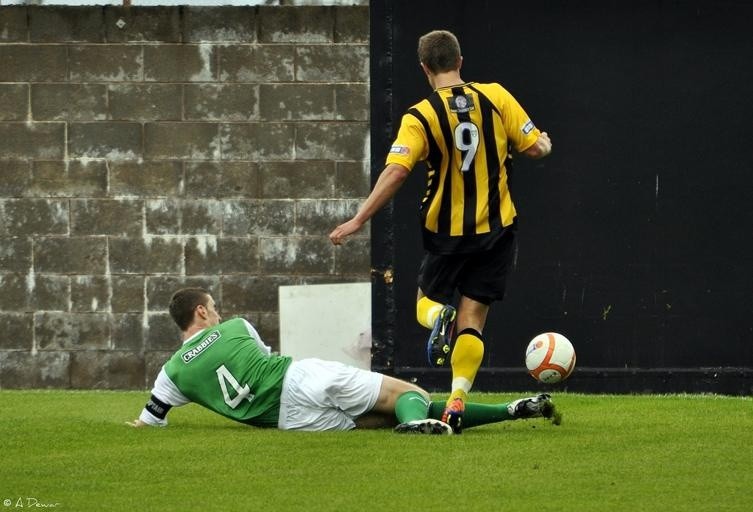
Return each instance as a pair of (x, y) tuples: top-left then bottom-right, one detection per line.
(426, 305), (456, 368)
(393, 409), (465, 437)
(507, 394), (552, 418)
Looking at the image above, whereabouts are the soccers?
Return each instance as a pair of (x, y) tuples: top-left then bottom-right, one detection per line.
(526, 333), (577, 384)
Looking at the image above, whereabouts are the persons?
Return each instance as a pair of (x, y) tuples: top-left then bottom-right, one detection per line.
(124, 287), (561, 433)
(329, 30), (556, 435)
